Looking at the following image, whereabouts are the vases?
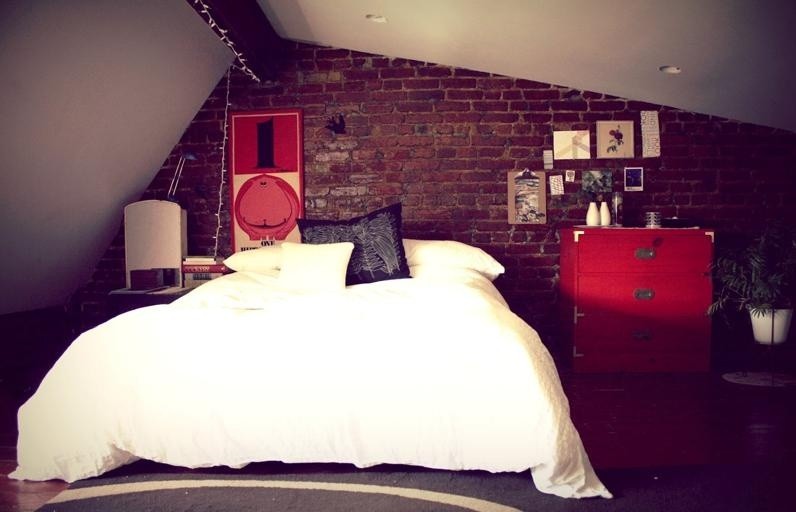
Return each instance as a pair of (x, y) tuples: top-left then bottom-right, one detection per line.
(585, 201), (612, 225)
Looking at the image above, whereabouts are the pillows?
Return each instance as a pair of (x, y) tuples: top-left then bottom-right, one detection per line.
(219, 201), (506, 285)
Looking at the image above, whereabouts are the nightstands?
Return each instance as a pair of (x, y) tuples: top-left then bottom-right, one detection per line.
(108, 287), (195, 319)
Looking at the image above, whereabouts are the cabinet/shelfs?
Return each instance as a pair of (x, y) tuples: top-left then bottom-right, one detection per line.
(558, 227), (715, 376)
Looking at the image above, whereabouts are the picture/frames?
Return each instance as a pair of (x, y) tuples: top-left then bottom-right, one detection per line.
(595, 121), (635, 160)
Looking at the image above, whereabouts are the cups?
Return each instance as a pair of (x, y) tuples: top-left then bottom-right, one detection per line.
(646, 211), (662, 228)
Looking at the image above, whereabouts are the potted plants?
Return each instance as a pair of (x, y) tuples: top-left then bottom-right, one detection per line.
(700, 216), (796, 345)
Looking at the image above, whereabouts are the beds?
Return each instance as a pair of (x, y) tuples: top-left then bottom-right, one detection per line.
(84, 271), (537, 471)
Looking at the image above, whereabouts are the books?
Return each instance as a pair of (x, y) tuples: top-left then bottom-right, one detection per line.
(181, 255), (227, 287)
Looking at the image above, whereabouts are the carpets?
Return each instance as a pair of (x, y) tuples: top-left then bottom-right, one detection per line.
(34, 472), (615, 512)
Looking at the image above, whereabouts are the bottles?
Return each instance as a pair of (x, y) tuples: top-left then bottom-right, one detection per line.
(585, 200), (611, 226)
(611, 192), (625, 227)
